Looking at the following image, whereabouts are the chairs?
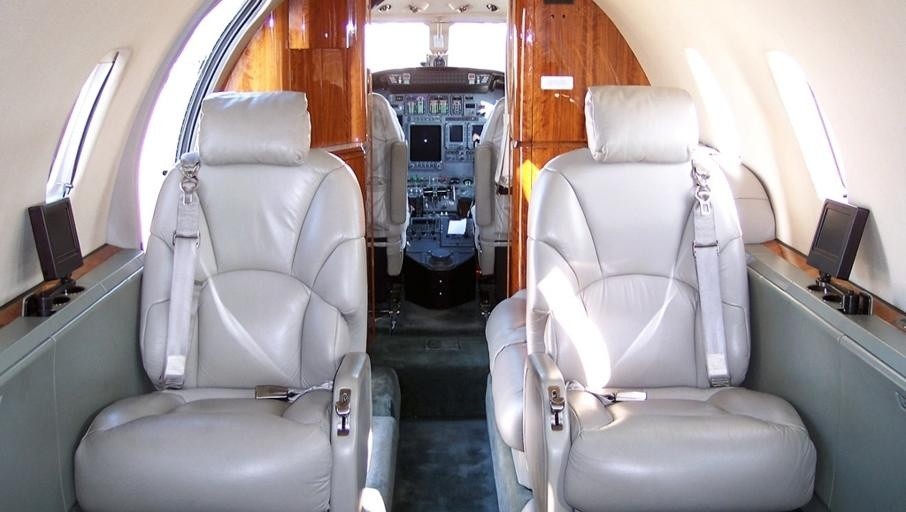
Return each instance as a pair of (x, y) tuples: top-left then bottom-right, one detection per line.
(73, 82), (371, 506)
(367, 92), (409, 330)
(472, 93), (517, 322)
(483, 92), (816, 510)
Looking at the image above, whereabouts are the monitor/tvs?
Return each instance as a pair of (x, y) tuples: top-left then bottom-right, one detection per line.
(27, 196), (84, 281)
(806, 197), (869, 282)
